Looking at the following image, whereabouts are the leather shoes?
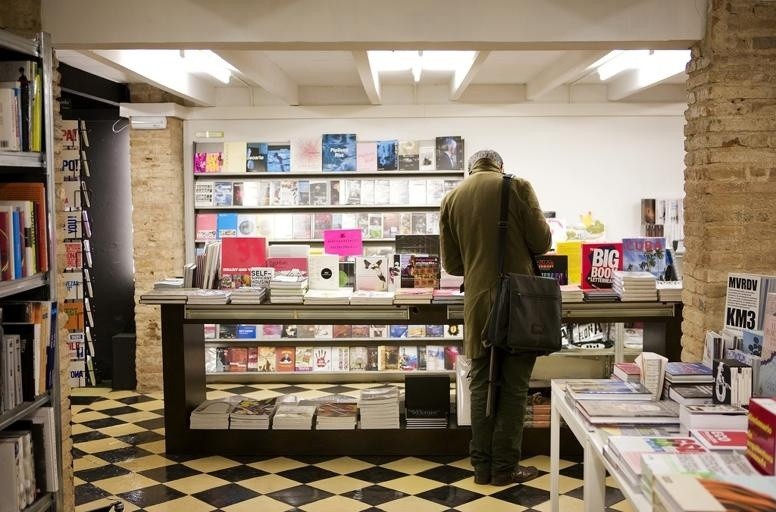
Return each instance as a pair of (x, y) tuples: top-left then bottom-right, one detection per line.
(475, 465), (539, 486)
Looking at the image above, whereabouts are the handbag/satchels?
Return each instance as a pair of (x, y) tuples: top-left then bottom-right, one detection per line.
(480, 275), (562, 353)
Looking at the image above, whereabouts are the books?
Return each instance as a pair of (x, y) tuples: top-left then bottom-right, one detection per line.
(1, 300), (58, 412)
(137, 234), (465, 305)
(1, 59), (43, 152)
(2, 406), (59, 511)
(192, 132), (465, 256)
(2, 182), (50, 280)
(62, 119), (96, 388)
(537, 197), (685, 351)
(191, 324), (465, 430)
(557, 273), (776, 512)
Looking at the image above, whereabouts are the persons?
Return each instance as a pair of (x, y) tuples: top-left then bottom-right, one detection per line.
(438, 149), (551, 486)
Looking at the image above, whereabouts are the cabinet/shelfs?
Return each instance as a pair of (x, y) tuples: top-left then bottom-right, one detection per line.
(0, 30), (63, 512)
(192, 139), (473, 384)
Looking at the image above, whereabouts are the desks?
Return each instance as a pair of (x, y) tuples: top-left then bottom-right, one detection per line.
(551, 378), (653, 512)
